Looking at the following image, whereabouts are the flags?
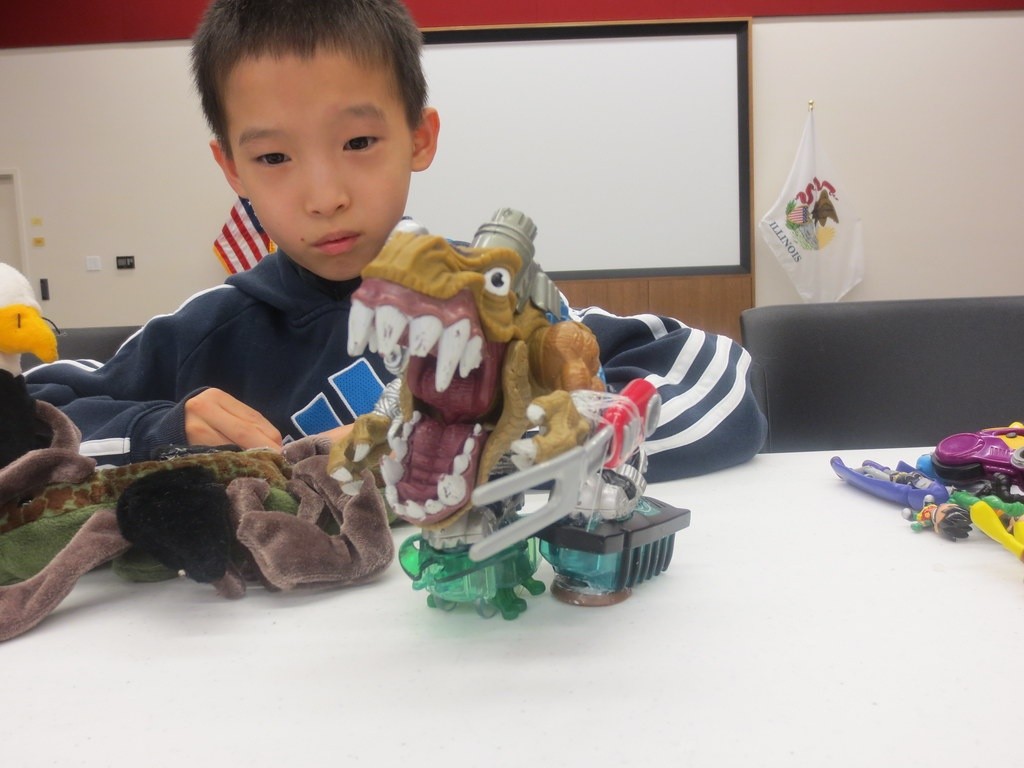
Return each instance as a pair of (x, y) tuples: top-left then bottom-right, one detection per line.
(212, 193), (275, 274)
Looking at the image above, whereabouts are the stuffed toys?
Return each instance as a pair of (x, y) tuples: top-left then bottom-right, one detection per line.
(0, 263), (395, 642)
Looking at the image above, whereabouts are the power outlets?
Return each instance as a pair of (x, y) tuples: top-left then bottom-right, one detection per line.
(86, 255), (101, 270)
(117, 256), (135, 269)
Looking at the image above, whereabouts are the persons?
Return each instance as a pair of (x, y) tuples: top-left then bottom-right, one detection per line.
(830, 457), (973, 543)
(0, 0), (768, 492)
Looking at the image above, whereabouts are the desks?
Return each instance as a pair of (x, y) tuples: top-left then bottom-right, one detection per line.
(0, 443), (1024, 768)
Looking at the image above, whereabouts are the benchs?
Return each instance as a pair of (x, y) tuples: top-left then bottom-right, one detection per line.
(739, 293), (1024, 453)
(19, 324), (143, 374)
